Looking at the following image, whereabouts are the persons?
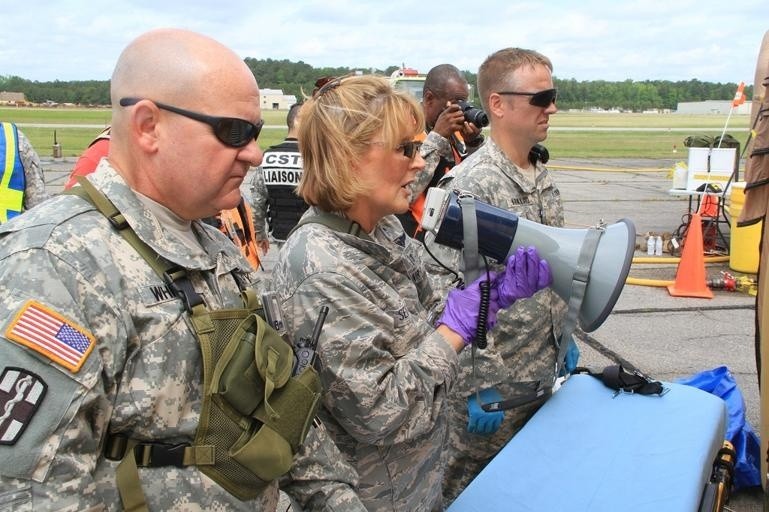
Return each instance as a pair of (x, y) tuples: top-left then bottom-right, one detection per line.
(394, 60), (490, 241)
(0, 120), (52, 230)
(252, 102), (311, 253)
(64, 124), (266, 274)
(0, 30), (366, 512)
(259, 74), (554, 512)
(420, 48), (583, 512)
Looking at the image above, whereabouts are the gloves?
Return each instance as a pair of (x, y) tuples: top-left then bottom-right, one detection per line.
(557, 334), (579, 376)
(435, 271), (499, 344)
(467, 388), (503, 433)
(497, 246), (552, 308)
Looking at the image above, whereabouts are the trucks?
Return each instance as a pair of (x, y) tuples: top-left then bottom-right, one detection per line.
(356, 68), (474, 113)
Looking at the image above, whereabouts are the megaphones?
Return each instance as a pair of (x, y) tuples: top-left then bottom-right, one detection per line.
(418, 187), (638, 332)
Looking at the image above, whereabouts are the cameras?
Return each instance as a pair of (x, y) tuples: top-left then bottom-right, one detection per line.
(451, 101), (488, 131)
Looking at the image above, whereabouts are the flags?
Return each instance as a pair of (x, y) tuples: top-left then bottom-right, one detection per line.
(4, 297), (96, 374)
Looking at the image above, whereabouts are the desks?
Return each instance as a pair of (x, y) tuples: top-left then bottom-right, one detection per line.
(667, 187), (733, 256)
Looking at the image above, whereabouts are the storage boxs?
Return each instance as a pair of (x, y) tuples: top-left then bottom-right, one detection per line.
(687, 145), (736, 171)
(688, 171), (733, 192)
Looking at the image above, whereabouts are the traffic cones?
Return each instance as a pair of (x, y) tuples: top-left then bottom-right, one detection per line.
(667, 215), (714, 301)
(697, 195), (720, 254)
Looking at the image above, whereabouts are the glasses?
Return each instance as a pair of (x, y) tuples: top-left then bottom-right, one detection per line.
(120, 99), (265, 148)
(365, 138), (423, 157)
(498, 88), (557, 107)
